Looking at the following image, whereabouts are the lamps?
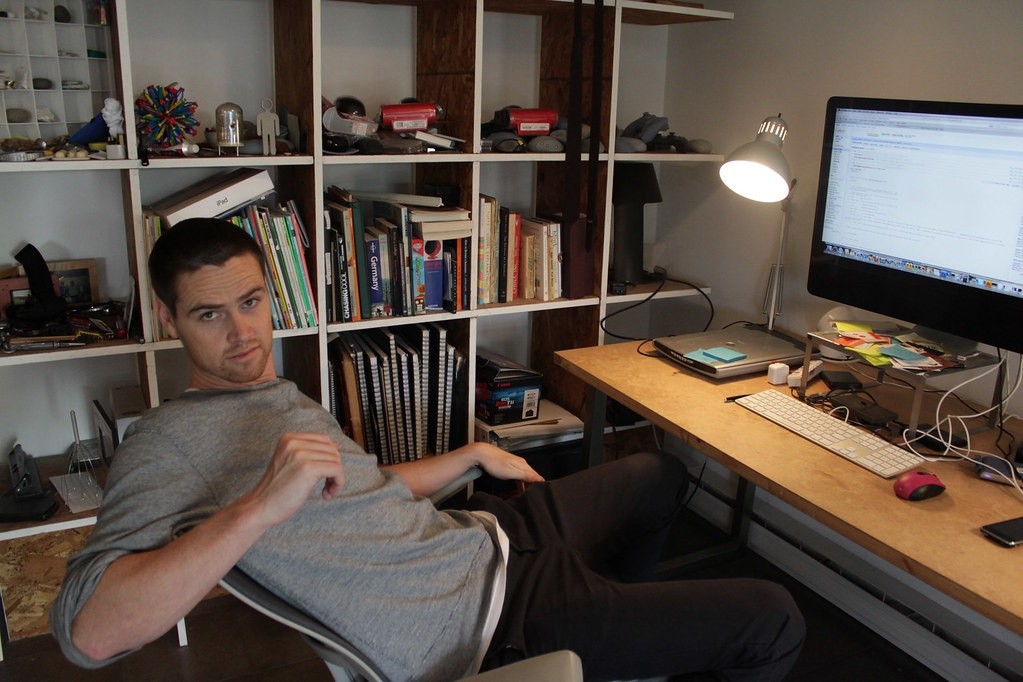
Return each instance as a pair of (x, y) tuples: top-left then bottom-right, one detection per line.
(719, 111), (799, 337)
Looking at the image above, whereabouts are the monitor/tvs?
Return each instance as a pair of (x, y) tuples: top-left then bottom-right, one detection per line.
(806, 97), (1023, 370)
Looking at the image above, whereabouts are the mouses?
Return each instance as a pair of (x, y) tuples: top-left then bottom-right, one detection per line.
(894, 471), (946, 501)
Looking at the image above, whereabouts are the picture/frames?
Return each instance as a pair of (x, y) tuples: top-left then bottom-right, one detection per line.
(16, 257), (100, 307)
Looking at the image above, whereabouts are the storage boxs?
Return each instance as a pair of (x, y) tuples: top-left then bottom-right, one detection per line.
(474, 396), (583, 451)
(475, 345), (544, 426)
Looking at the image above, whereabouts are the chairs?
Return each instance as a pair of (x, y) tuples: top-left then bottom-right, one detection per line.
(171, 528), (583, 682)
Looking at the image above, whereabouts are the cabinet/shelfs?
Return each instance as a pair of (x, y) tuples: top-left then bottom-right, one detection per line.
(0, 0), (735, 644)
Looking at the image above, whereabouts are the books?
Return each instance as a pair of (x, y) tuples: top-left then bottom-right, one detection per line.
(142, 184), (586, 465)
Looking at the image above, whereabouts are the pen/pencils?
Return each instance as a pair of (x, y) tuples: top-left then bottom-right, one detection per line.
(724, 394), (754, 402)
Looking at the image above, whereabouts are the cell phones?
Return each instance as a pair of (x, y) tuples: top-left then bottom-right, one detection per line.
(981, 517), (1023, 547)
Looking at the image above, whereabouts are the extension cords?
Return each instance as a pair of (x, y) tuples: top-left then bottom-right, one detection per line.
(788, 360), (823, 388)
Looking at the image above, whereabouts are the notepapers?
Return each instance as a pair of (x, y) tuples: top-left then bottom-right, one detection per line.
(703, 346), (747, 364)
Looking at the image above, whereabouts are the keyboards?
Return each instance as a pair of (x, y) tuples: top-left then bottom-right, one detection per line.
(735, 389), (924, 478)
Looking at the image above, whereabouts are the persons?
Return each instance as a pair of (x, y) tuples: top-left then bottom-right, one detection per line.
(49, 218), (806, 682)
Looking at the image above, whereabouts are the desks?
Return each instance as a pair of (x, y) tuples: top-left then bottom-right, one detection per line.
(553, 326), (1023, 682)
